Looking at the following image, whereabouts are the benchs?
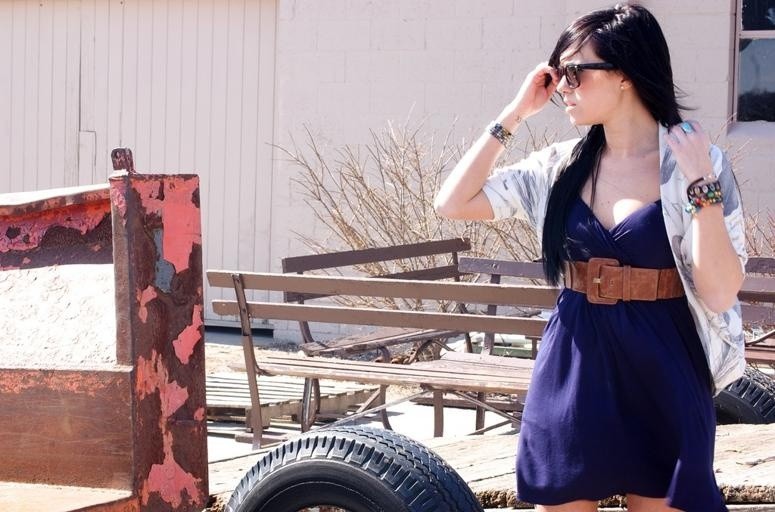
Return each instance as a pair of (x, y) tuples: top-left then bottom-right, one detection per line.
(422, 255), (565, 437)
(523, 255), (773, 375)
(207, 270), (564, 439)
(280, 235), (473, 435)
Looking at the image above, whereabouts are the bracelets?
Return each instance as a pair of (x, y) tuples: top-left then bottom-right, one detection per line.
(485, 120), (515, 150)
(686, 175), (725, 214)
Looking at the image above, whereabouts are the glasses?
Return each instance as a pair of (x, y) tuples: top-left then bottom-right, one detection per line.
(556, 62), (620, 90)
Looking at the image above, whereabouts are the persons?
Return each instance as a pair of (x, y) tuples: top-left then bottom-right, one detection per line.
(434, 2), (755, 510)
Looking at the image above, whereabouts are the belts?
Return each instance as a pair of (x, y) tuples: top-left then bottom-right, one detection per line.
(561, 256), (687, 306)
